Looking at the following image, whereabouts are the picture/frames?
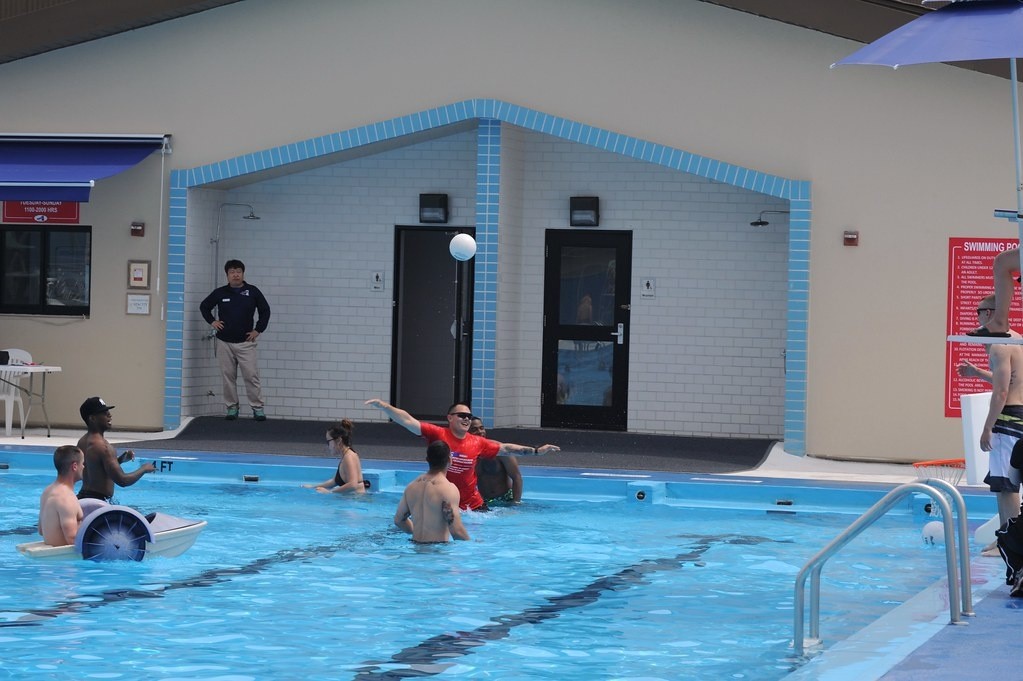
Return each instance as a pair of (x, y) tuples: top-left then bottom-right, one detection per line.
(126, 292), (151, 316)
(127, 259), (151, 289)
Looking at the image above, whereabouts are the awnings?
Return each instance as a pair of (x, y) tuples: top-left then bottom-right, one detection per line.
(0, 135), (163, 202)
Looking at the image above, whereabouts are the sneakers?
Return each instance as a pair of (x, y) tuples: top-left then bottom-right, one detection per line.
(225, 408), (238, 419)
(254, 410), (266, 421)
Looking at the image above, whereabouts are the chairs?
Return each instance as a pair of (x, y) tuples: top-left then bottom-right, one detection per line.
(0, 349), (32, 436)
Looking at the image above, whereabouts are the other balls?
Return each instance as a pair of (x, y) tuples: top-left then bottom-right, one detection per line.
(451, 234), (476, 261)
(922, 521), (944, 545)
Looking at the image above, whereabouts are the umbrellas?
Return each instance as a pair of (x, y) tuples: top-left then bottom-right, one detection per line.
(831, 0), (1021, 280)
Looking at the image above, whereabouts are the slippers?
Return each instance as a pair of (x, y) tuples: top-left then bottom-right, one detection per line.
(965, 325), (1012, 337)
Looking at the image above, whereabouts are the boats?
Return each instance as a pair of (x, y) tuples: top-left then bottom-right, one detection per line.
(17, 499), (209, 566)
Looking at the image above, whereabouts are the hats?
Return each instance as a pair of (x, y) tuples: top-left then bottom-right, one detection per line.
(80, 397), (115, 419)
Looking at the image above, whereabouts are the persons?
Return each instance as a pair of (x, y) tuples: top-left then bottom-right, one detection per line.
(301, 419), (365, 495)
(364, 398), (561, 512)
(77, 397), (159, 502)
(468, 416), (522, 507)
(954, 295), (1023, 557)
(576, 295), (593, 324)
(966, 247), (1023, 338)
(200, 260), (270, 421)
(394, 441), (470, 545)
(995, 437), (1023, 598)
(47, 446), (84, 547)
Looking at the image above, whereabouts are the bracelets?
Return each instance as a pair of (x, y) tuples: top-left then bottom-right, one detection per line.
(534, 445), (539, 455)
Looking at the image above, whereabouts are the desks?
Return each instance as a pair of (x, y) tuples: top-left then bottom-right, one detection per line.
(0, 365), (62, 439)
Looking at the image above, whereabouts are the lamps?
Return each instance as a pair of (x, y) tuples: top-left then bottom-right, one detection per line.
(570, 195), (599, 227)
(419, 193), (448, 224)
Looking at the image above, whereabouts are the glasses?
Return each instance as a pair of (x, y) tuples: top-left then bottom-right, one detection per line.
(977, 308), (996, 315)
(451, 412), (472, 421)
(327, 438), (338, 446)
